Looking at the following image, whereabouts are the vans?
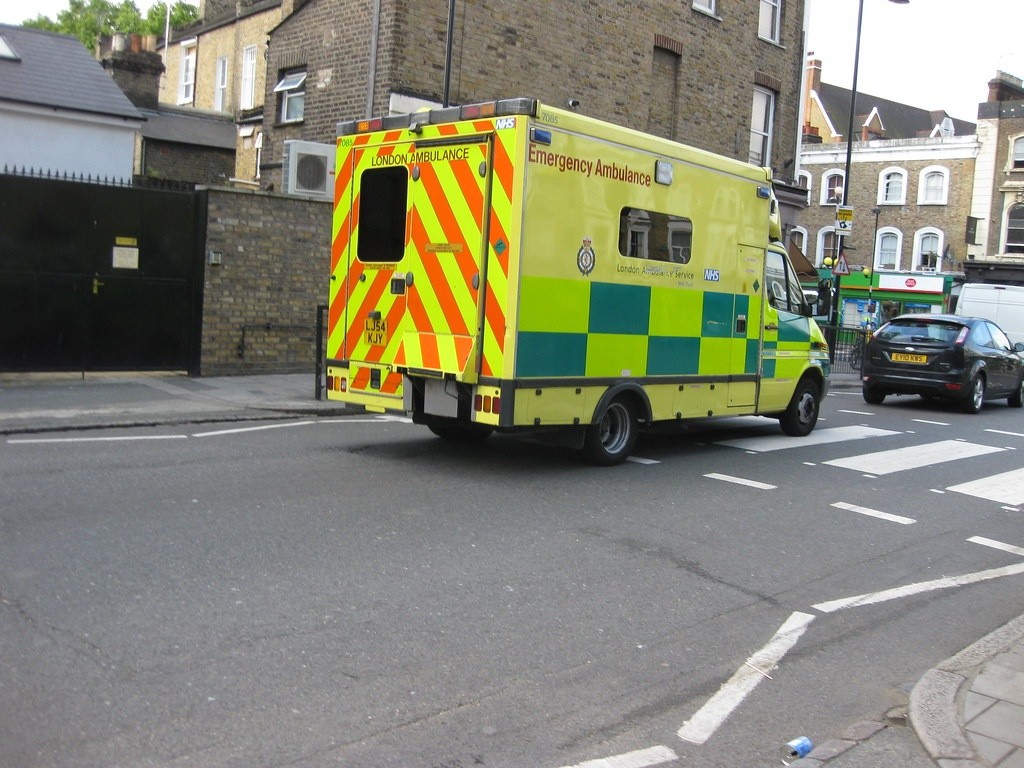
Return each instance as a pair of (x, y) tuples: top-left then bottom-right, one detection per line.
(954, 284), (1024, 355)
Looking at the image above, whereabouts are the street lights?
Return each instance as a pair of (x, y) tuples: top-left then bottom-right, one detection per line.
(861, 208), (883, 343)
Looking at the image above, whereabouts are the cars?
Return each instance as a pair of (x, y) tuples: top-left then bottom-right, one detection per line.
(860, 310), (1024, 408)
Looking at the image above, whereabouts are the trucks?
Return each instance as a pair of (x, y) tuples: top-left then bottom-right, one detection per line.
(323, 94), (839, 469)
